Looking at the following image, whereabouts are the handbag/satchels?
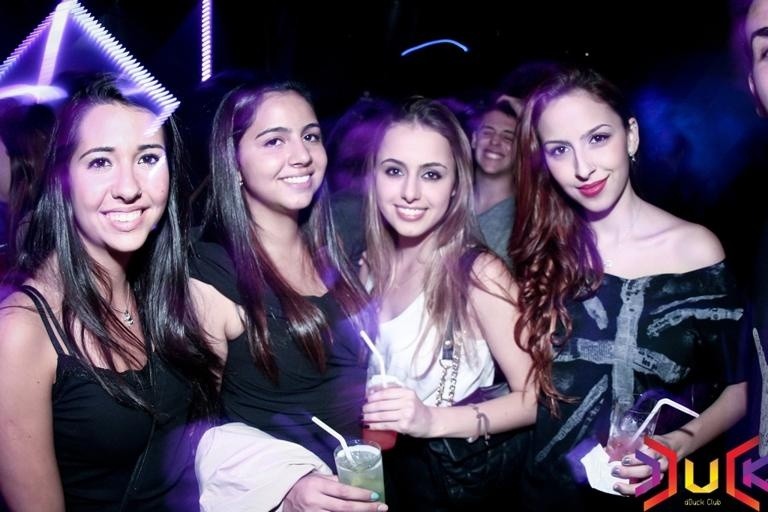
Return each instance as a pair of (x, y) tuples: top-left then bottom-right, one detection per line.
(425, 381), (534, 507)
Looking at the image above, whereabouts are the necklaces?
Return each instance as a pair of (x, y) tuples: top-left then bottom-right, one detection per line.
(108, 280), (136, 331)
(392, 247), (439, 291)
(602, 202), (643, 272)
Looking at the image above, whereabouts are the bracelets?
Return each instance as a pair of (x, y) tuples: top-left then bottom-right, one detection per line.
(465, 395), (492, 450)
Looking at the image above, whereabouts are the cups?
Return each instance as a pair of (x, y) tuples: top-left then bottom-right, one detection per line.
(604, 389), (662, 465)
(331, 439), (388, 504)
(360, 363), (406, 453)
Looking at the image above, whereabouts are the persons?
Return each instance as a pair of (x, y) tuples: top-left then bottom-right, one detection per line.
(717, 2), (768, 511)
(0, 87), (528, 283)
(348, 93), (546, 511)
(0, 75), (228, 511)
(148, 74), (392, 512)
(505, 61), (750, 512)
